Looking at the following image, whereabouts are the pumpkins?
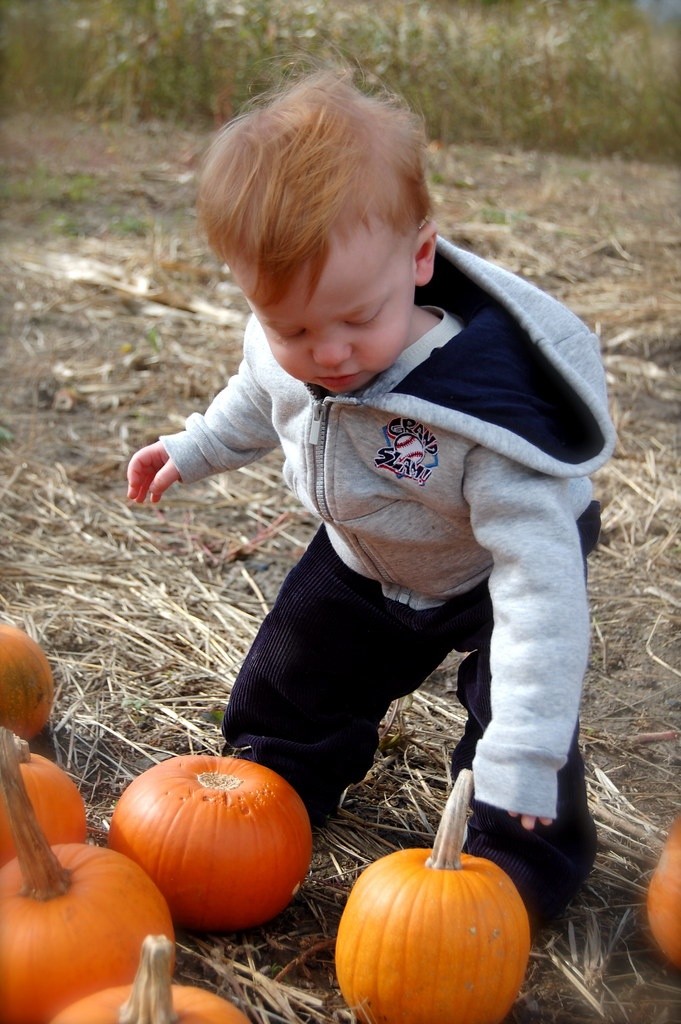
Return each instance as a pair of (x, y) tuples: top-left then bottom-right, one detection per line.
(0, 620), (681, 1024)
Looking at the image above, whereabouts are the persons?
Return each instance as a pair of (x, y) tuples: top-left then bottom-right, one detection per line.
(126, 68), (618, 920)
